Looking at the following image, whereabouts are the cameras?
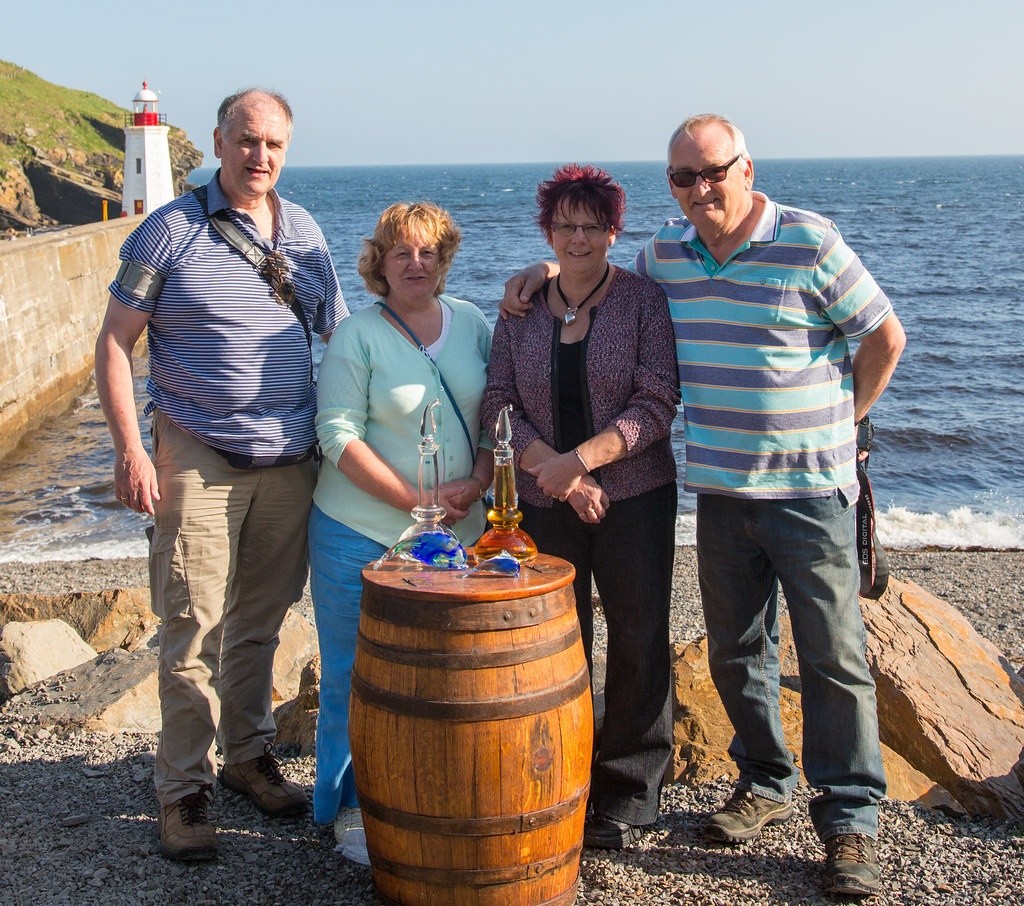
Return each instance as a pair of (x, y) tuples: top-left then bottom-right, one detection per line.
(855, 416), (871, 451)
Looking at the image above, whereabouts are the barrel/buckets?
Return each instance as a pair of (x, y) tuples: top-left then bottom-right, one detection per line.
(347, 552), (595, 906)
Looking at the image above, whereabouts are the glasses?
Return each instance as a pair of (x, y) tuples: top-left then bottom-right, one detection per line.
(548, 220), (611, 238)
(669, 154), (741, 188)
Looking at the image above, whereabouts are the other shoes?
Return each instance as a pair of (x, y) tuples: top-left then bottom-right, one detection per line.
(582, 814), (648, 849)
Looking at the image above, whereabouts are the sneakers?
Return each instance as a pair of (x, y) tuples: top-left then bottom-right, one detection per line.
(824, 834), (880, 894)
(219, 751), (308, 818)
(158, 792), (217, 862)
(704, 789), (793, 844)
(334, 806), (364, 845)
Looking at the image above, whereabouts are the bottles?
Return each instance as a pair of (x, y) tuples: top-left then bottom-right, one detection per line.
(399, 399), (459, 560)
(472, 404), (539, 568)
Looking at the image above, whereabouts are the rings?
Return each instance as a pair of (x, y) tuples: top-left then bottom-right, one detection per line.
(120, 495), (127, 500)
(552, 494), (558, 498)
(579, 513), (586, 516)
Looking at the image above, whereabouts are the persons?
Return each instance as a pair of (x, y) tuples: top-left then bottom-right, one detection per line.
(479, 164), (682, 851)
(306, 203), (493, 865)
(498, 114), (907, 896)
(95, 88), (349, 865)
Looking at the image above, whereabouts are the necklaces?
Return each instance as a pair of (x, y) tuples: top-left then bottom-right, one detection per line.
(556, 262), (610, 325)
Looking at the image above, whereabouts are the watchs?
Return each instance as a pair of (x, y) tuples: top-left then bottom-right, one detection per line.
(472, 476), (484, 502)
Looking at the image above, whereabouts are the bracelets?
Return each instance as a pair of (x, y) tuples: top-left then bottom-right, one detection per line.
(574, 448), (590, 472)
